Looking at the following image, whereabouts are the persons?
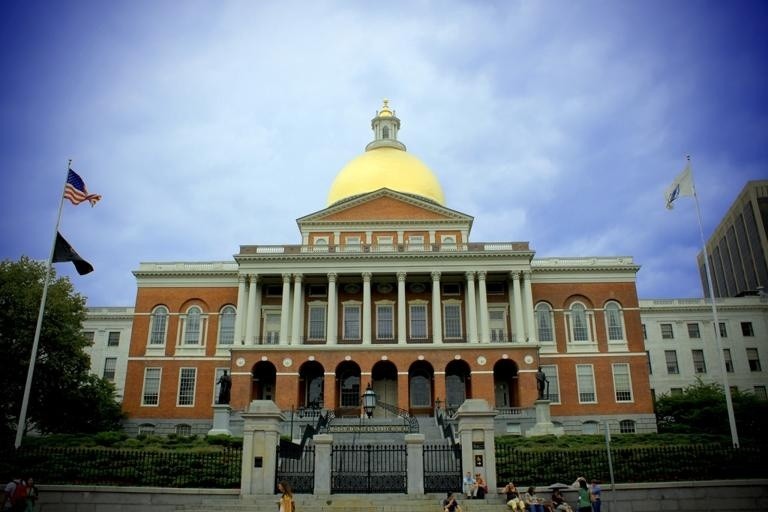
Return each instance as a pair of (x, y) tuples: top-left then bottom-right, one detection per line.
(278, 480), (292, 512)
(443, 471), (601, 512)
(216, 370), (232, 404)
(535, 367), (545, 400)
(25, 477), (39, 512)
(0, 474), (27, 512)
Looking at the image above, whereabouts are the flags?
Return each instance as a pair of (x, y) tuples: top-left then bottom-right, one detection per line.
(64, 169), (103, 208)
(52, 231), (93, 275)
(666, 166), (695, 209)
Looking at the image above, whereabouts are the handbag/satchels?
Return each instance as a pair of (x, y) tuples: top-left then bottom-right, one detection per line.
(276, 502), (295, 512)
